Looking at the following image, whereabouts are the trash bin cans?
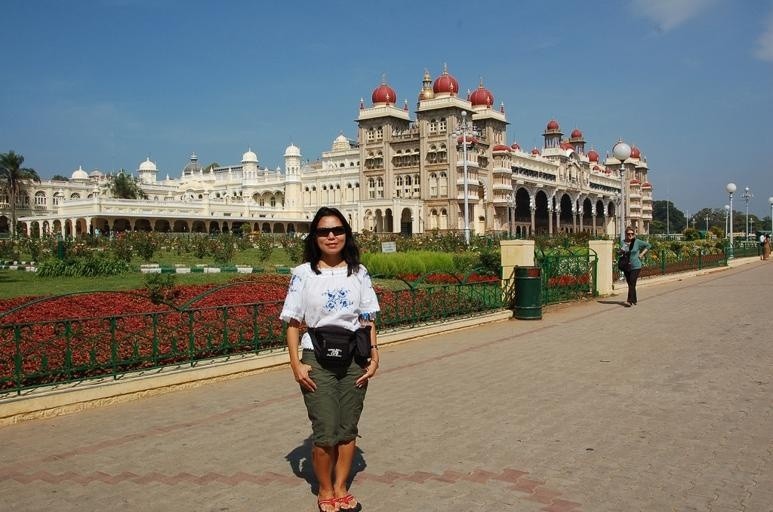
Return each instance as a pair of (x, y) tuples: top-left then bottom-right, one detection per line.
(513, 265), (542, 321)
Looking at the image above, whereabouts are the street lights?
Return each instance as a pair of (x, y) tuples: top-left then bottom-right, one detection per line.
(723, 182), (773, 260)
(612, 140), (632, 245)
(451, 111), (478, 247)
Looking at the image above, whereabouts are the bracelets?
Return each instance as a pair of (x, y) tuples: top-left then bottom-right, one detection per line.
(370, 359), (379, 370)
(369, 344), (378, 350)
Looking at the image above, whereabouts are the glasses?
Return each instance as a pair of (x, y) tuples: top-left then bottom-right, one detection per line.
(317, 226), (345, 237)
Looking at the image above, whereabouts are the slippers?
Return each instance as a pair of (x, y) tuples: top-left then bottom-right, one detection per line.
(318, 489), (362, 512)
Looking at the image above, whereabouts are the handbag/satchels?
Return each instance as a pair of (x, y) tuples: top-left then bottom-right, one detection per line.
(618, 252), (631, 271)
(308, 326), (372, 376)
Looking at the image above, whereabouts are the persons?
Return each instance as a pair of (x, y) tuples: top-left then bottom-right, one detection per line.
(614, 225), (651, 306)
(276, 205), (382, 511)
(759, 232), (766, 261)
(762, 234), (770, 260)
